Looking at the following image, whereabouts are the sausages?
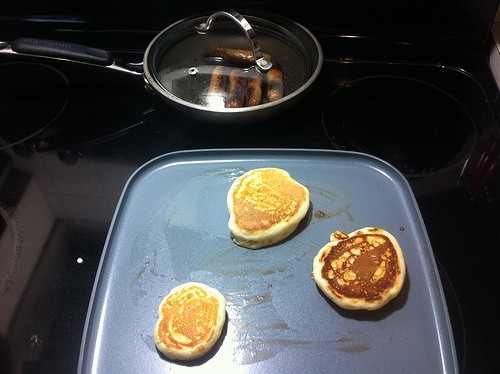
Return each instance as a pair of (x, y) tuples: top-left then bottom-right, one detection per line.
(207, 48), (285, 108)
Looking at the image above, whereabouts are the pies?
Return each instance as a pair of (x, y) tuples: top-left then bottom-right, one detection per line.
(227, 168), (309, 250)
(312, 227), (406, 312)
(153, 282), (226, 362)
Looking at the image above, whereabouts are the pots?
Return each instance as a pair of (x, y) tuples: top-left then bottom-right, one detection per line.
(0, 8), (324, 126)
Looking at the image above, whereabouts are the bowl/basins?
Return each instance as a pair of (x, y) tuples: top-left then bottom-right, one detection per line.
(1, 60), (76, 159)
(320, 72), (478, 195)
